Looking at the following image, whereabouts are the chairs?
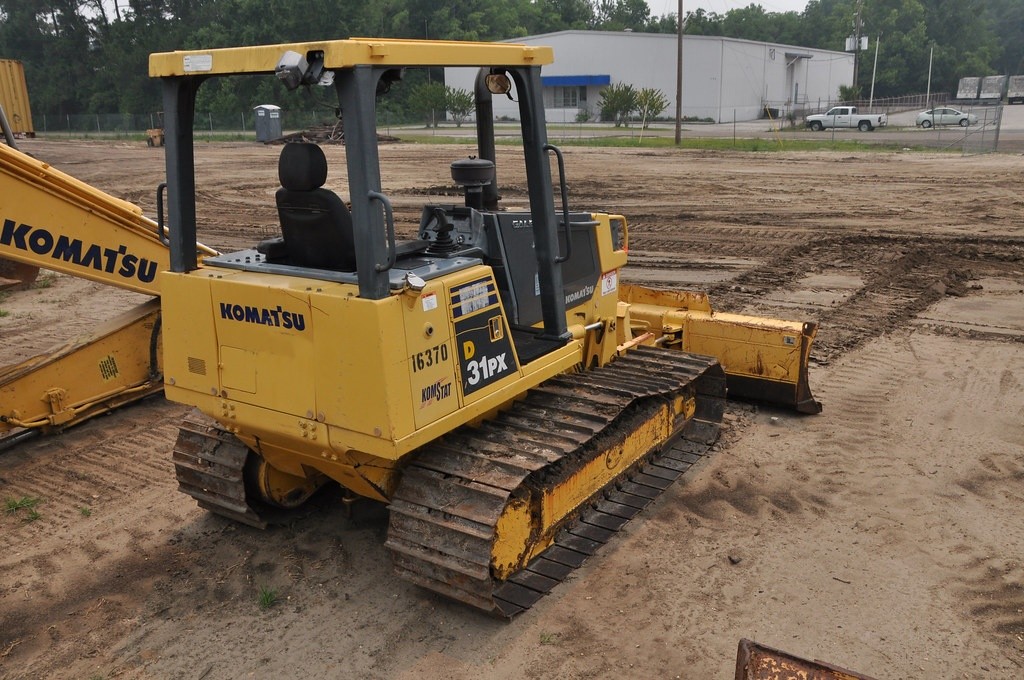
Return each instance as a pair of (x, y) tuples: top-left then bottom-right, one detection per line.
(275, 141), (429, 273)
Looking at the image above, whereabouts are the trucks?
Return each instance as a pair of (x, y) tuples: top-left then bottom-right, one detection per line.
(148, 37), (823, 624)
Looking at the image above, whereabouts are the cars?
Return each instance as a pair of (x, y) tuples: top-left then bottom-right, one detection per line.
(916, 108), (979, 128)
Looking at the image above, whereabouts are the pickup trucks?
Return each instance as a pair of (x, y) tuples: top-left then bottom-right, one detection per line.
(806, 106), (887, 132)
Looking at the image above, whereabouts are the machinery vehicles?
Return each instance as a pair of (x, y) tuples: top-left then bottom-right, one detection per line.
(1, 101), (226, 454)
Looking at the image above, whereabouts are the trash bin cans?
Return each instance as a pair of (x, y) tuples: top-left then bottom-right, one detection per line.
(253, 105), (281, 142)
